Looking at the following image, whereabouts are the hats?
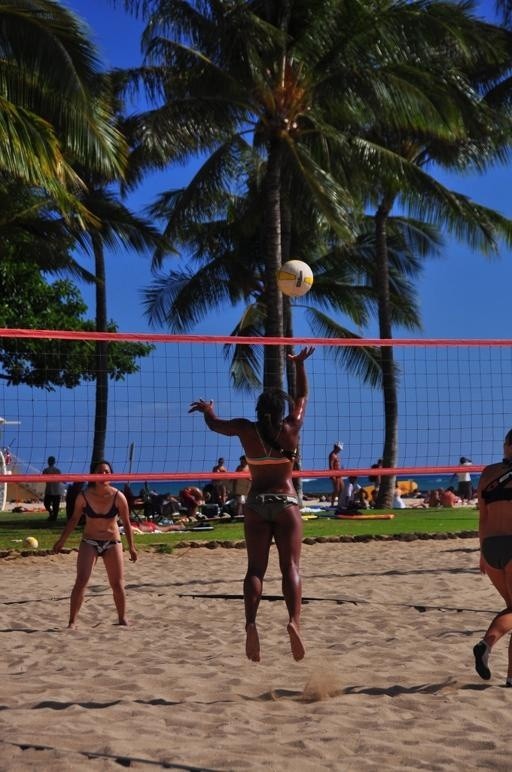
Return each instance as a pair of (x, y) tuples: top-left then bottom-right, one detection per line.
(335, 442), (343, 451)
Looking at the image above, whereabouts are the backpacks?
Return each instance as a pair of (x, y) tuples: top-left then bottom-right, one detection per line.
(369, 464), (379, 481)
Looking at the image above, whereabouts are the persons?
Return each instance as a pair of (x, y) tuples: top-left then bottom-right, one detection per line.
(210, 457), (229, 505)
(186, 344), (317, 662)
(372, 459), (384, 504)
(427, 487), (443, 507)
(53, 460), (139, 629)
(42, 455), (64, 522)
(182, 486), (212, 518)
(233, 455), (253, 498)
(471, 427), (511, 693)
(326, 440), (346, 507)
(455, 457), (473, 505)
(389, 482), (408, 509)
(340, 475), (369, 508)
(439, 486), (457, 508)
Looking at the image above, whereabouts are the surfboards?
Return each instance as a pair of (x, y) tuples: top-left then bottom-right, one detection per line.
(362, 480), (418, 502)
(302, 515), (318, 520)
(322, 513), (396, 518)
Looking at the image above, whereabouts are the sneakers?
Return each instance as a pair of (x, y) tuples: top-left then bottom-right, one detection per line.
(473, 640), (493, 681)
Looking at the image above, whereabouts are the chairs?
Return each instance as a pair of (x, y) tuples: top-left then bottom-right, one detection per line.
(126, 495), (154, 522)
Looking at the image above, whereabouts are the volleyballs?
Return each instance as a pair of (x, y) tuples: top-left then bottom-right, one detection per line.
(23, 536), (39, 550)
(275, 258), (314, 297)
